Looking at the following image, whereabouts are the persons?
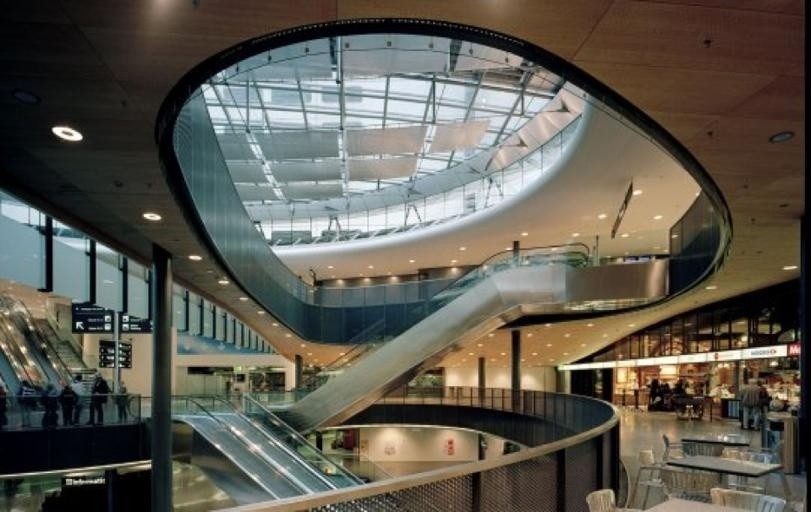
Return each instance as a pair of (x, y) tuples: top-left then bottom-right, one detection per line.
(86, 371), (109, 425)
(58, 386), (79, 425)
(72, 374), (84, 425)
(740, 380), (766, 432)
(116, 380), (129, 420)
(756, 381), (770, 428)
(14, 379), (39, 426)
(1, 375), (10, 426)
(41, 383), (60, 426)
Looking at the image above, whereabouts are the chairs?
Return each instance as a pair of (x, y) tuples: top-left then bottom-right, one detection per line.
(585, 429), (788, 511)
